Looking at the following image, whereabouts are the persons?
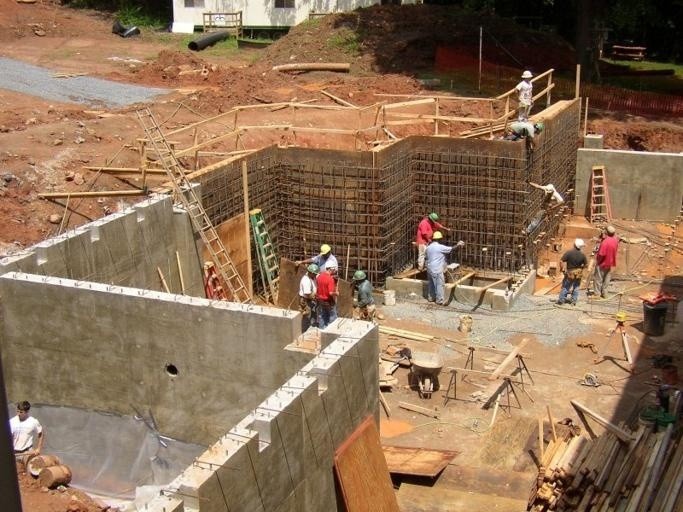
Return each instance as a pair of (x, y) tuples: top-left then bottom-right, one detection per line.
(294, 243), (338, 274)
(588, 225), (619, 302)
(298, 263), (319, 329)
(416, 213), (451, 271)
(498, 121), (543, 154)
(514, 70), (534, 121)
(314, 261), (339, 329)
(522, 180), (565, 236)
(422, 231), (466, 307)
(349, 271), (376, 321)
(8, 399), (45, 466)
(556, 236), (588, 305)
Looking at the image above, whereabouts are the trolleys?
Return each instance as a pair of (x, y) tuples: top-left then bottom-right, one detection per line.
(409, 350), (443, 398)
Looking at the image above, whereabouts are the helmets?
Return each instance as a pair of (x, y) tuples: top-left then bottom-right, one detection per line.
(433, 231), (443, 239)
(352, 271), (367, 280)
(429, 213), (439, 222)
(320, 244), (331, 254)
(606, 227), (616, 235)
(537, 122), (544, 132)
(575, 238), (585, 251)
(521, 71), (533, 78)
(546, 184), (554, 194)
(307, 264), (320, 275)
(325, 262), (336, 269)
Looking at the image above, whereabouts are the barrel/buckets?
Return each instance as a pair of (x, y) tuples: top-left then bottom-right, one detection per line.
(662, 364), (678, 384)
(27, 455), (59, 475)
(383, 290), (396, 305)
(459, 316), (472, 332)
(39, 465), (72, 488)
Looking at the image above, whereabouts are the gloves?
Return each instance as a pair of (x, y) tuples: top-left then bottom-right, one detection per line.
(457, 240), (464, 248)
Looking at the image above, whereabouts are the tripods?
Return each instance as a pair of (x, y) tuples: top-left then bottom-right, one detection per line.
(596, 312), (633, 367)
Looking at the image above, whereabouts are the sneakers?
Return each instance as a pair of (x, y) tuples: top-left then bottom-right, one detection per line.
(418, 266), (424, 270)
(558, 300), (577, 306)
(592, 293), (609, 299)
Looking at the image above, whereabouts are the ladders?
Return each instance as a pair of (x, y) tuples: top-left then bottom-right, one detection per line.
(590, 166), (613, 225)
(249, 209), (280, 305)
(135, 105), (253, 304)
